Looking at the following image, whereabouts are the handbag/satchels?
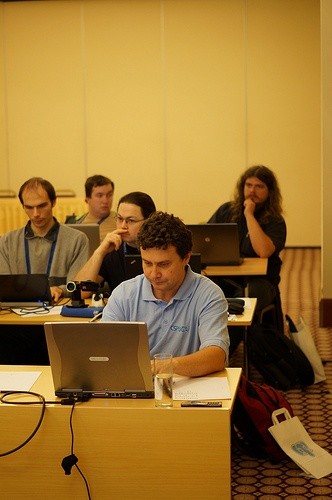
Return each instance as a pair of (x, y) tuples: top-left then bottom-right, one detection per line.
(268, 407), (332, 480)
(284, 313), (327, 385)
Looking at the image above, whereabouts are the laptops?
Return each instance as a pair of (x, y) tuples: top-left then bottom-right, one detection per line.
(43, 321), (154, 399)
(0, 274), (54, 306)
(186, 224), (243, 266)
(125, 255), (144, 280)
(64, 224), (101, 261)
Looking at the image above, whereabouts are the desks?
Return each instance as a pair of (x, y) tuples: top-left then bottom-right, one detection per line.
(0, 297), (257, 380)
(0, 365), (243, 500)
(201, 257), (268, 297)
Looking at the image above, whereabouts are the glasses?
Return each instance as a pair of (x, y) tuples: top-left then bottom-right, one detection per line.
(113, 213), (147, 225)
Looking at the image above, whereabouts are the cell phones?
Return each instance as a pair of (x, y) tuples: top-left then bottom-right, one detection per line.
(181, 401), (222, 408)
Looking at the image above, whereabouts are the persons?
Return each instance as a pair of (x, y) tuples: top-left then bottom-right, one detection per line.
(0, 178), (89, 366)
(208, 166), (286, 354)
(68, 175), (117, 241)
(73, 192), (156, 298)
(101, 214), (229, 377)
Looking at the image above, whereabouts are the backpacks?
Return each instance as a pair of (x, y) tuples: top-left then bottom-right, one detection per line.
(243, 326), (315, 391)
(233, 374), (294, 464)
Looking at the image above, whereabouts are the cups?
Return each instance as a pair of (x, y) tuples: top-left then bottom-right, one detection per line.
(153, 353), (173, 408)
